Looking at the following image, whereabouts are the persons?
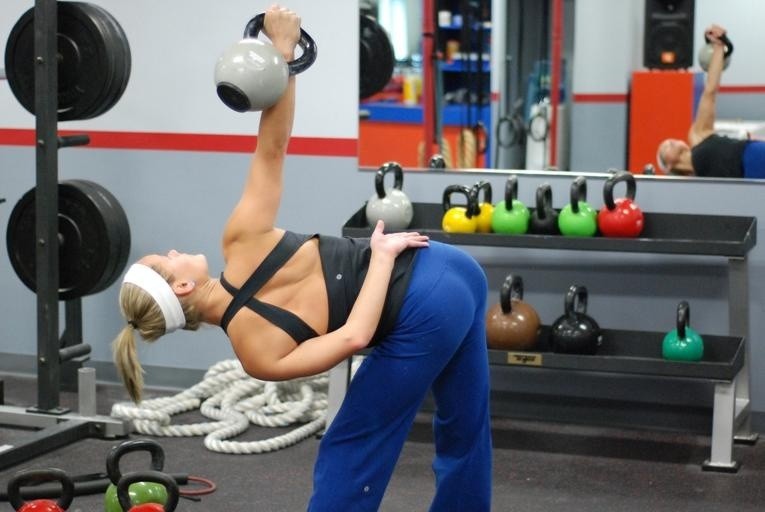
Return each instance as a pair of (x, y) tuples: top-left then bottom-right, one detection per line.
(112, 6), (494, 511)
(655, 25), (765, 178)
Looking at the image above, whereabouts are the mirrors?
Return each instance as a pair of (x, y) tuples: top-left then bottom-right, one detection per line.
(359, 1), (765, 184)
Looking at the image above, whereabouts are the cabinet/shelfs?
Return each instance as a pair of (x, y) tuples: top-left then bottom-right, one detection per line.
(316, 201), (760, 472)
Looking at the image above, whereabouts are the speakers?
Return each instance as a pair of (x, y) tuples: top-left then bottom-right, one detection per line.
(644, 1), (694, 72)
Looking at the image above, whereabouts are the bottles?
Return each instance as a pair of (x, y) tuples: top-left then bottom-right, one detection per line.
(403, 55), (423, 106)
